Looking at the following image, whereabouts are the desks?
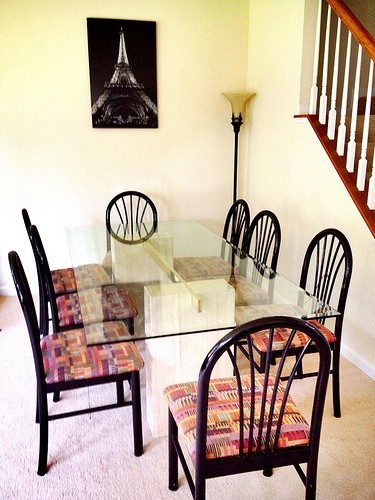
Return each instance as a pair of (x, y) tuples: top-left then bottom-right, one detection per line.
(104, 215), (342, 440)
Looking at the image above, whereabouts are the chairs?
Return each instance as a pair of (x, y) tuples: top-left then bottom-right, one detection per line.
(162, 315), (332, 500)
(7, 251), (146, 477)
(232, 228), (353, 418)
(21, 207), (138, 403)
(187, 210), (282, 376)
(170, 200), (250, 315)
(104, 191), (159, 282)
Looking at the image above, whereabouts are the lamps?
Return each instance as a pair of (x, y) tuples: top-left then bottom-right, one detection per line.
(218, 91), (258, 307)
